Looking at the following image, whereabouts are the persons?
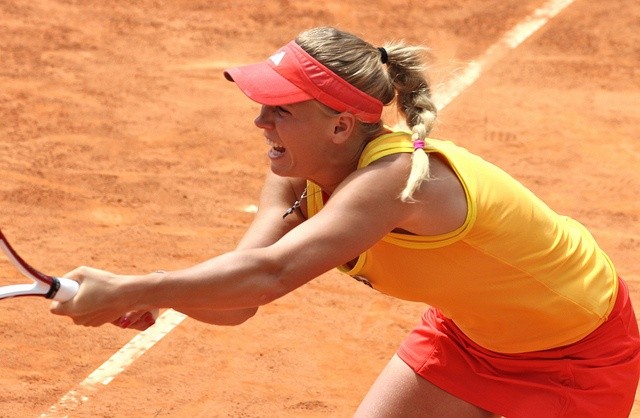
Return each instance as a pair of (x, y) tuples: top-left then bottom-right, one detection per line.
(49, 25), (640, 418)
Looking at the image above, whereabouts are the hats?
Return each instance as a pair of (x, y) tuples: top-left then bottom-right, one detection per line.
(224, 41), (382, 123)
